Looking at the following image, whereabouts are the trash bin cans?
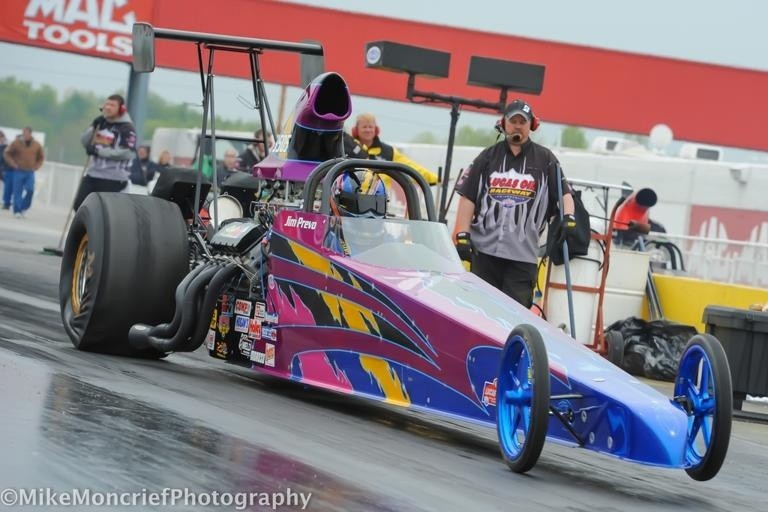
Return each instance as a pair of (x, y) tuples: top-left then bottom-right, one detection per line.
(703, 305), (768, 411)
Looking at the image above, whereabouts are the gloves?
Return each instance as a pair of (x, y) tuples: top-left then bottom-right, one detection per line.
(552, 214), (576, 260)
(86, 144), (95, 155)
(456, 231), (478, 261)
(93, 114), (103, 125)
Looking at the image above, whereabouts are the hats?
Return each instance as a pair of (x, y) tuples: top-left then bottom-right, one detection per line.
(503, 100), (532, 121)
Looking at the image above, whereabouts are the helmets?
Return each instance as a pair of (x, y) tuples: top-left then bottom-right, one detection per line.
(330, 168), (388, 217)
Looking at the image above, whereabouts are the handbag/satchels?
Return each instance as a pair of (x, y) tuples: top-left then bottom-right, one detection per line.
(603, 316), (697, 381)
(547, 188), (591, 266)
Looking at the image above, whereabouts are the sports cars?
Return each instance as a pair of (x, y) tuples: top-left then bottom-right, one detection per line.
(59, 20), (732, 481)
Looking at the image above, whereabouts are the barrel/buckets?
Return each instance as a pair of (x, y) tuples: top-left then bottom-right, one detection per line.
(594, 249), (650, 331)
(546, 248), (601, 345)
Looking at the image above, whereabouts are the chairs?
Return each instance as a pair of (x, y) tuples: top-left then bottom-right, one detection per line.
(303, 158), (423, 220)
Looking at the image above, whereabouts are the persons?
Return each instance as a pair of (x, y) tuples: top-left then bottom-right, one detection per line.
(73, 94), (136, 212)
(154, 149), (171, 178)
(612, 188), (665, 249)
(454, 98), (576, 309)
(0, 131), (14, 209)
(210, 148), (238, 183)
(123, 146), (167, 196)
(3, 127), (45, 218)
(343, 113), (443, 203)
(238, 128), (275, 174)
